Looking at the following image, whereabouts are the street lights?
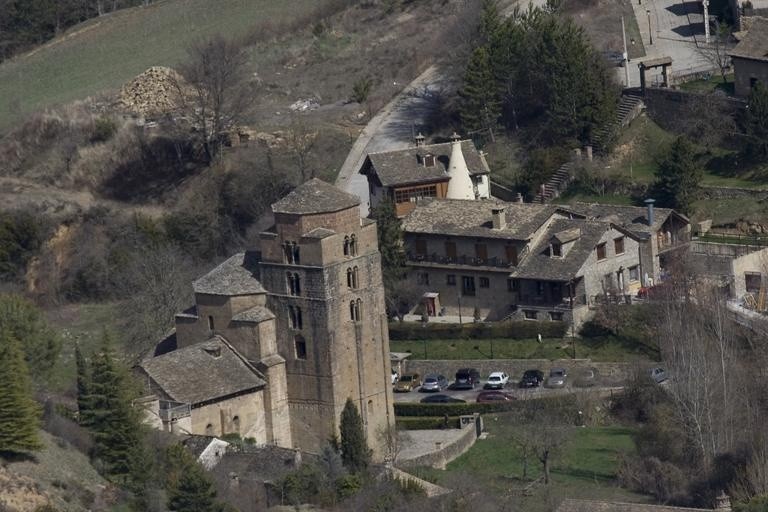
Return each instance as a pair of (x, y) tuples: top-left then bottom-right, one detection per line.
(458, 294), (462, 323)
(645, 10), (654, 45)
(566, 272), (576, 359)
(488, 321), (493, 357)
(421, 320), (427, 358)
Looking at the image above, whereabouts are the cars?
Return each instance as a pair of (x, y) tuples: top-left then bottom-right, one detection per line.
(392, 367), (664, 402)
(638, 284), (661, 298)
